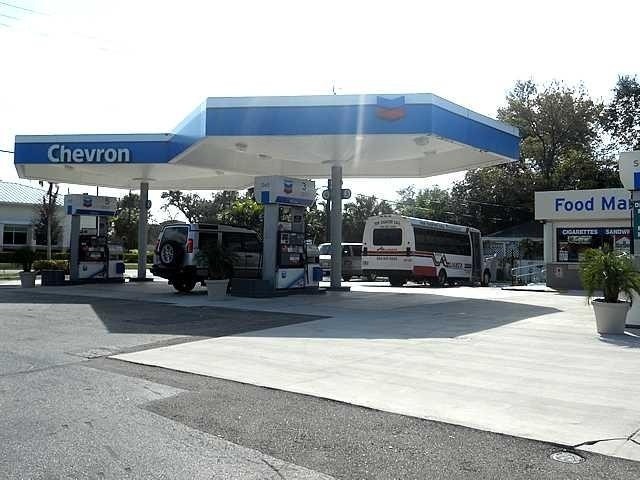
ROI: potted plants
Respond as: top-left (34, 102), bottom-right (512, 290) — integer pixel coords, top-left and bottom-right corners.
top-left (191, 239), bottom-right (241, 300)
top-left (10, 246), bottom-right (39, 288)
top-left (578, 247), bottom-right (640, 335)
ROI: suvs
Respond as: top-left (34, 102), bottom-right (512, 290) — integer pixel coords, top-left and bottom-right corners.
top-left (152, 222), bottom-right (265, 292)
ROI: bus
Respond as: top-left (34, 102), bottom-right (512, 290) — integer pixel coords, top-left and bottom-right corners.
top-left (361, 213), bottom-right (491, 287)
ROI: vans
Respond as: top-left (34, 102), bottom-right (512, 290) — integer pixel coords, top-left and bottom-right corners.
top-left (317, 243), bottom-right (379, 282)
